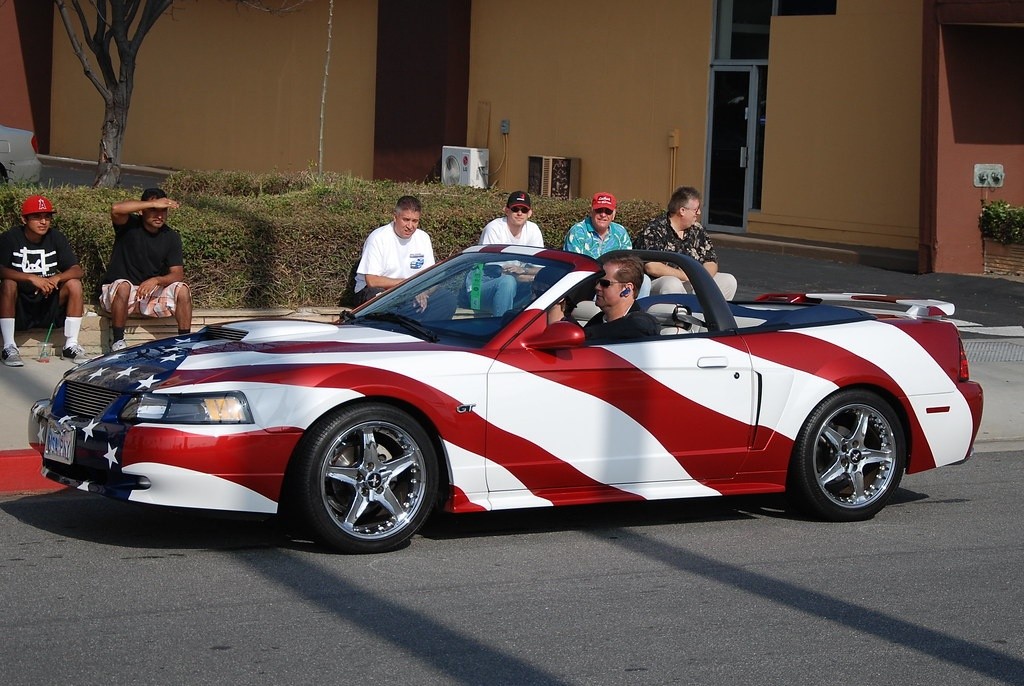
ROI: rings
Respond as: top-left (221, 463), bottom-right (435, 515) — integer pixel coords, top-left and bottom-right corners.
top-left (169, 202), bottom-right (172, 205)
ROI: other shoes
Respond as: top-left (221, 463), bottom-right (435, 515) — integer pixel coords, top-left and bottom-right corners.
top-left (111, 340), bottom-right (126, 352)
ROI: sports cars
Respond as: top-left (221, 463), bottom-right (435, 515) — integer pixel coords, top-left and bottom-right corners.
top-left (27, 242), bottom-right (985, 556)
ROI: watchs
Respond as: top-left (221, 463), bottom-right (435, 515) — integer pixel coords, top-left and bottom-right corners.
top-left (522, 267), bottom-right (529, 275)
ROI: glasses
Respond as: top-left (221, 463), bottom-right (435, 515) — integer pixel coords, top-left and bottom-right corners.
top-left (682, 206), bottom-right (700, 215)
top-left (511, 206), bottom-right (530, 213)
top-left (529, 288), bottom-right (544, 297)
top-left (595, 208), bottom-right (615, 215)
top-left (596, 278), bottom-right (630, 288)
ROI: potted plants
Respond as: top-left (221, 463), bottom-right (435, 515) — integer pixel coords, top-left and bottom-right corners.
top-left (977, 198), bottom-right (1024, 276)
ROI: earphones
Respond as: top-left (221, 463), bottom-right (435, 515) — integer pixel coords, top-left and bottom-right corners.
top-left (620, 287), bottom-right (631, 297)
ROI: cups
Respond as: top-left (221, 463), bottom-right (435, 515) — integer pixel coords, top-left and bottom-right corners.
top-left (36, 342), bottom-right (54, 363)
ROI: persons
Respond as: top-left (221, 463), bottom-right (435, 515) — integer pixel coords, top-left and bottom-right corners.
top-left (353, 196), bottom-right (457, 321)
top-left (559, 251), bottom-right (663, 344)
top-left (562, 191), bottom-right (632, 262)
top-left (632, 186), bottom-right (738, 311)
top-left (0, 194), bottom-right (91, 366)
top-left (466, 190), bottom-right (546, 317)
top-left (501, 262), bottom-right (582, 328)
top-left (98, 187), bottom-right (192, 351)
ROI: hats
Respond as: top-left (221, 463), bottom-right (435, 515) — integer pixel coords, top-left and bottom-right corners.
top-left (21, 196), bottom-right (57, 214)
top-left (536, 267), bottom-right (578, 309)
top-left (507, 191), bottom-right (530, 209)
top-left (592, 192), bottom-right (617, 211)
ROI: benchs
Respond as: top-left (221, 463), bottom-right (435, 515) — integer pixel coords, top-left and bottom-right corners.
top-left (571, 301), bottom-right (767, 337)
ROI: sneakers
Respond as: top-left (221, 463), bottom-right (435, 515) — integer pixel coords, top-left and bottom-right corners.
top-left (61, 345), bottom-right (89, 365)
top-left (1, 345), bottom-right (24, 365)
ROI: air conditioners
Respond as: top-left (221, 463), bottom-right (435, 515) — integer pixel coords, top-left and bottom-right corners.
top-left (441, 146), bottom-right (490, 189)
